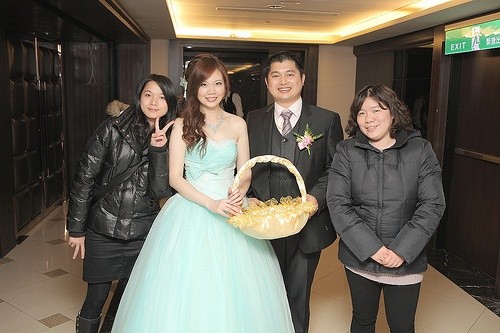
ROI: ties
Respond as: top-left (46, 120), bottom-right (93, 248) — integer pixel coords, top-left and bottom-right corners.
top-left (280, 110), bottom-right (293, 137)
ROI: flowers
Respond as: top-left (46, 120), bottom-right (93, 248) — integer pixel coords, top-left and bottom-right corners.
top-left (293, 123), bottom-right (325, 156)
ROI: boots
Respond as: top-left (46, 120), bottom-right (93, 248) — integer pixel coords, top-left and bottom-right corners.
top-left (76, 311), bottom-right (115, 333)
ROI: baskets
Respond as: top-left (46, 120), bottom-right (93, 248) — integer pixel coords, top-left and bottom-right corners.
top-left (226, 154), bottom-right (317, 240)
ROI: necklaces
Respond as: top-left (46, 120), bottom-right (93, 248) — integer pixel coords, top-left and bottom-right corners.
top-left (199, 105), bottom-right (225, 134)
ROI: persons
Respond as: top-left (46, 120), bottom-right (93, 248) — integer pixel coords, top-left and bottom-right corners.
top-left (168, 53), bottom-right (252, 332)
top-left (65, 72), bottom-right (180, 333)
top-left (243, 51), bottom-right (345, 333)
top-left (326, 83), bottom-right (446, 333)
